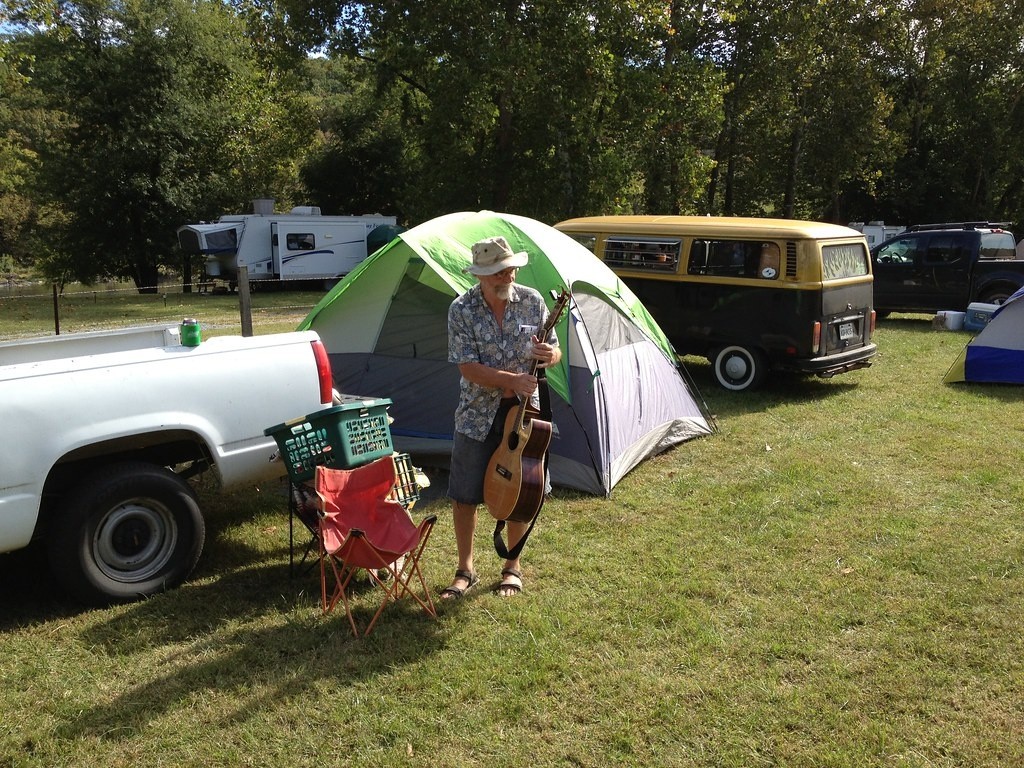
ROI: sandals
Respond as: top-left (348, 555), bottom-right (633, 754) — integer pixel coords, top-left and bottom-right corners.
top-left (440, 569), bottom-right (480, 601)
top-left (496, 567), bottom-right (522, 597)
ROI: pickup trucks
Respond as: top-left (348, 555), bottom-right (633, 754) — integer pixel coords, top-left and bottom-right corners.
top-left (869, 222), bottom-right (1024, 321)
top-left (0, 318), bottom-right (346, 605)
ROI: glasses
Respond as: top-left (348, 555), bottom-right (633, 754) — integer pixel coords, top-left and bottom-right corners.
top-left (494, 267), bottom-right (521, 280)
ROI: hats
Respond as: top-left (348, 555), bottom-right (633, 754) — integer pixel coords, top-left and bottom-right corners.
top-left (462, 237), bottom-right (528, 277)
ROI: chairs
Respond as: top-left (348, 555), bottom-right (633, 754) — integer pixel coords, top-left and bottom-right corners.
top-left (315, 456), bottom-right (438, 636)
top-left (278, 474), bottom-right (358, 584)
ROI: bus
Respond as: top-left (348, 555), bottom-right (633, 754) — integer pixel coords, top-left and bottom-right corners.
top-left (551, 213), bottom-right (877, 394)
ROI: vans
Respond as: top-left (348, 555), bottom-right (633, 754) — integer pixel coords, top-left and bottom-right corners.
top-left (177, 197), bottom-right (399, 297)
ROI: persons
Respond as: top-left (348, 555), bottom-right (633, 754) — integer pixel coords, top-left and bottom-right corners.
top-left (440, 236), bottom-right (562, 601)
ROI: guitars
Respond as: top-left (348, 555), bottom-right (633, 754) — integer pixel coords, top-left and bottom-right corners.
top-left (482, 285), bottom-right (573, 525)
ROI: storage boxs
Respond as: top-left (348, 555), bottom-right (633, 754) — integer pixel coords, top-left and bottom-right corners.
top-left (937, 311), bottom-right (966, 330)
top-left (963, 303), bottom-right (1001, 330)
top-left (262, 397), bottom-right (395, 483)
top-left (385, 454), bottom-right (421, 505)
